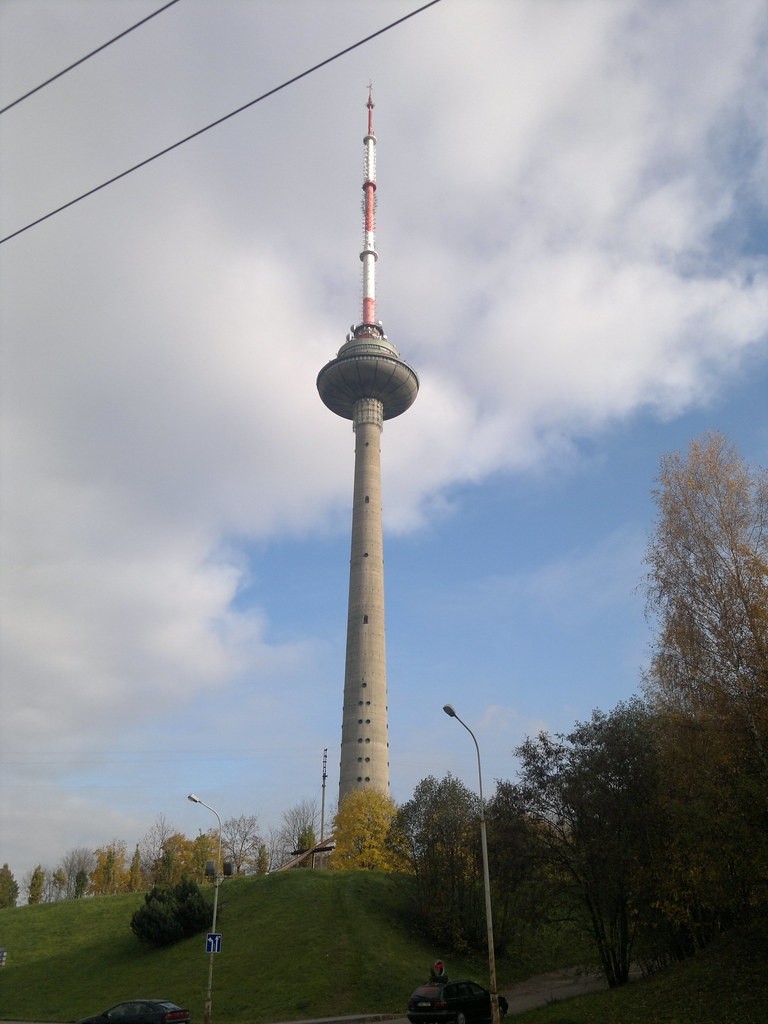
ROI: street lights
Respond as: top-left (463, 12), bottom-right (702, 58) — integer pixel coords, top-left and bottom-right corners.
top-left (443, 704), bottom-right (499, 1024)
top-left (188, 794), bottom-right (222, 1024)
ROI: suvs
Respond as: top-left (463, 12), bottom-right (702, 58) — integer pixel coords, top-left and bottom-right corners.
top-left (408, 975), bottom-right (509, 1024)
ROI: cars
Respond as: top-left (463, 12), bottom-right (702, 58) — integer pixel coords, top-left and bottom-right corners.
top-left (75, 999), bottom-right (191, 1024)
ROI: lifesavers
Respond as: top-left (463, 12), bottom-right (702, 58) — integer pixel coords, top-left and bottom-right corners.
top-left (430, 959), bottom-right (444, 976)
